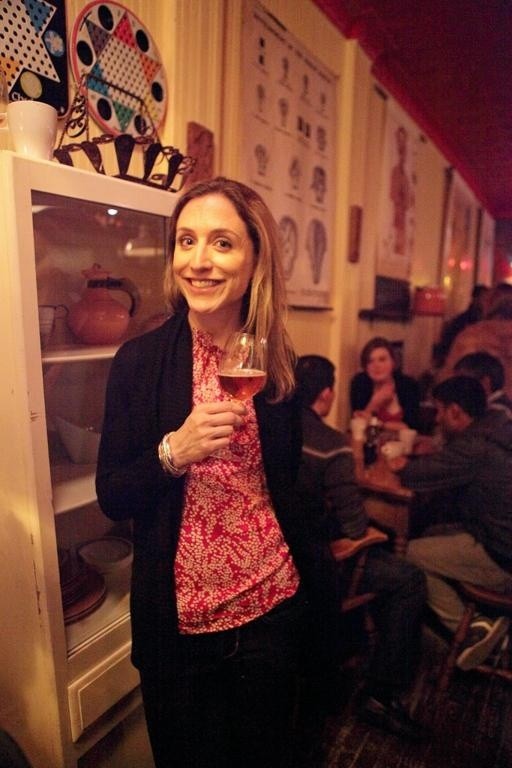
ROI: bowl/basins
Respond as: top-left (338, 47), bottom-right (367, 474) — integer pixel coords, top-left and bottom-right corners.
top-left (56, 414), bottom-right (101, 461)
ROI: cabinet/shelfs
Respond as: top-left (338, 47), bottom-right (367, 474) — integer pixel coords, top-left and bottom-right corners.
top-left (0, 149), bottom-right (180, 768)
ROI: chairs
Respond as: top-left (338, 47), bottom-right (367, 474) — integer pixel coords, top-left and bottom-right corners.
top-left (312, 527), bottom-right (389, 655)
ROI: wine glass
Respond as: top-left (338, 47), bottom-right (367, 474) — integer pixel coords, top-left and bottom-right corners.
top-left (207, 331), bottom-right (269, 466)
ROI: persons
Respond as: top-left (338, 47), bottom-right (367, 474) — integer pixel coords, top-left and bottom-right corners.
top-left (95, 179), bottom-right (312, 765)
top-left (293, 280), bottom-right (510, 691)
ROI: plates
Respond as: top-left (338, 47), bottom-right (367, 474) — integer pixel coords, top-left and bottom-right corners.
top-left (55, 536), bottom-right (133, 626)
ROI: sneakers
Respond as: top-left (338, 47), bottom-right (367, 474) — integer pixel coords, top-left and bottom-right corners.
top-left (455, 614), bottom-right (509, 671)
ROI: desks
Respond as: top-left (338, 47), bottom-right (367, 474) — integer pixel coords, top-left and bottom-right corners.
top-left (347, 469), bottom-right (457, 685)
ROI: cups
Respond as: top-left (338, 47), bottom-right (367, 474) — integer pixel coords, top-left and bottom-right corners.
top-left (2, 100), bottom-right (61, 163)
top-left (37, 300), bottom-right (69, 357)
top-left (348, 411), bottom-right (420, 483)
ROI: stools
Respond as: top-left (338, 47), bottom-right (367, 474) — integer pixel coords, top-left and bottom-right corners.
top-left (435, 581), bottom-right (512, 703)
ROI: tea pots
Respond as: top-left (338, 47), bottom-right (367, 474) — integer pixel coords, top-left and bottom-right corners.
top-left (69, 262), bottom-right (143, 348)
